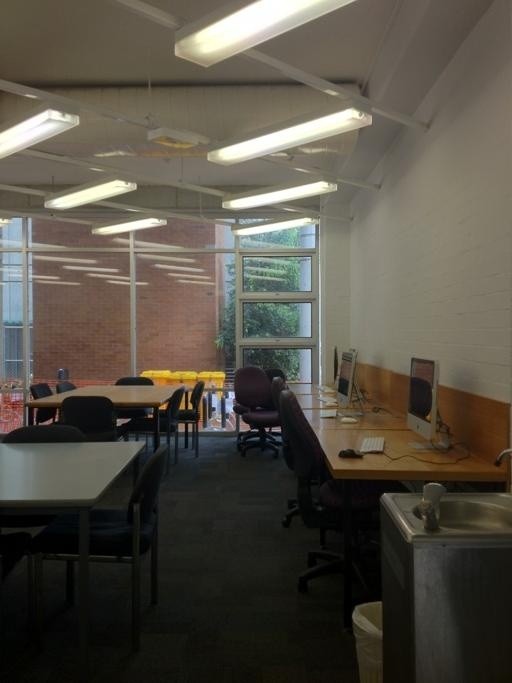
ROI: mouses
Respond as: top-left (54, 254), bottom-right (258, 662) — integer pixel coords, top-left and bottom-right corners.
top-left (325, 389), bottom-right (335, 394)
top-left (338, 448), bottom-right (362, 459)
top-left (326, 402), bottom-right (338, 407)
top-left (341, 417), bottom-right (358, 424)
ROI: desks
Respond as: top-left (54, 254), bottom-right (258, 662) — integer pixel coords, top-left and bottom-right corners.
top-left (1, 441), bottom-right (149, 655)
top-left (286, 384), bottom-right (506, 481)
top-left (28, 384), bottom-right (183, 449)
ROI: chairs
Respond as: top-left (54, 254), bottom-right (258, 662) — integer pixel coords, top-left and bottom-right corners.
top-left (238, 368), bottom-right (285, 443)
top-left (233, 366), bottom-right (280, 458)
top-left (33, 443), bottom-right (169, 649)
top-left (115, 376), bottom-right (153, 386)
top-left (290, 420), bottom-right (380, 634)
top-left (124, 386), bottom-right (185, 465)
top-left (3, 425), bottom-right (85, 443)
top-left (278, 389), bottom-right (312, 528)
top-left (163, 381), bottom-right (206, 458)
top-left (56, 381), bottom-right (76, 393)
top-left (60, 395), bottom-right (117, 441)
top-left (30, 383), bottom-right (58, 425)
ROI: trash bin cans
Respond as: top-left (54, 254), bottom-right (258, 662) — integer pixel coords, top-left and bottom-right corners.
top-left (197, 370), bottom-right (225, 421)
top-left (168, 371), bottom-right (197, 410)
top-left (352, 601), bottom-right (383, 683)
top-left (140, 369), bottom-right (169, 410)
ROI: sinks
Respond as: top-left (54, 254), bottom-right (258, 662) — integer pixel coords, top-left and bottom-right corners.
top-left (379, 492), bottom-right (512, 550)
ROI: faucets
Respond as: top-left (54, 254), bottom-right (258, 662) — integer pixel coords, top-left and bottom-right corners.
top-left (418, 481), bottom-right (447, 531)
top-left (493, 448), bottom-right (512, 468)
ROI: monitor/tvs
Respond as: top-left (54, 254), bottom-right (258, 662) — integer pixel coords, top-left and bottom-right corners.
top-left (337, 352), bottom-right (357, 406)
top-left (406, 356), bottom-right (440, 442)
top-left (332, 345), bottom-right (338, 384)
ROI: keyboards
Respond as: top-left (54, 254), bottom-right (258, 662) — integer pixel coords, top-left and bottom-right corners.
top-left (316, 396), bottom-right (337, 403)
top-left (316, 385), bottom-right (331, 390)
top-left (361, 436), bottom-right (384, 453)
top-left (320, 408), bottom-right (337, 419)
